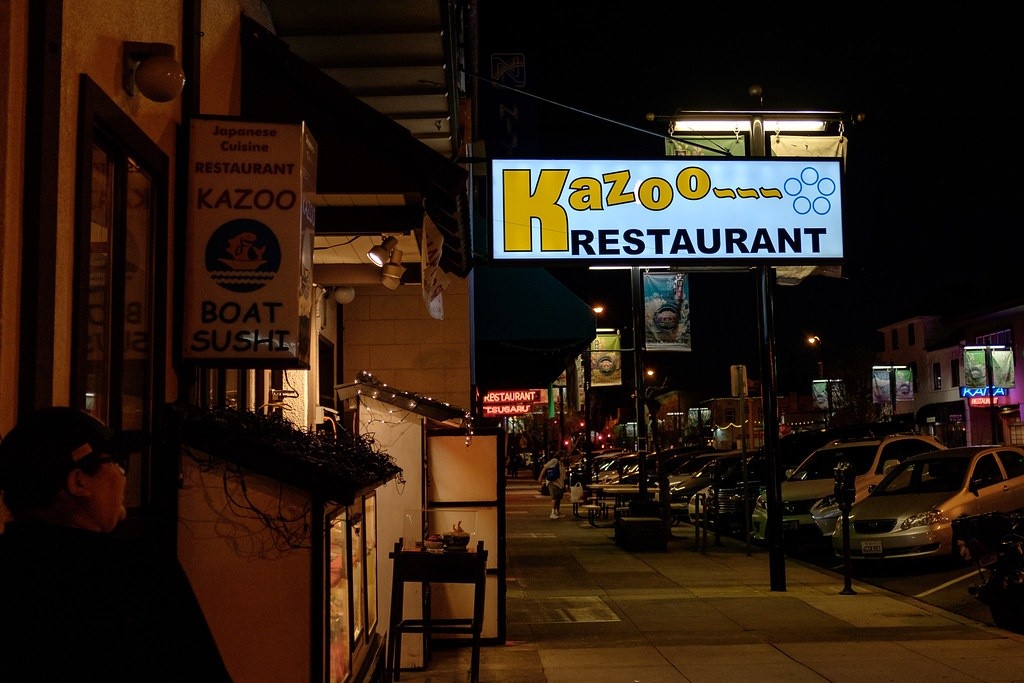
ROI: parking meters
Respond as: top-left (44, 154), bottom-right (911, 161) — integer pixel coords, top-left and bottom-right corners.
top-left (832, 459), bottom-right (857, 595)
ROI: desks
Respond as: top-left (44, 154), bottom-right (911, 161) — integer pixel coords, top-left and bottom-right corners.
top-left (586, 484), bottom-right (661, 527)
top-left (382, 538), bottom-right (488, 683)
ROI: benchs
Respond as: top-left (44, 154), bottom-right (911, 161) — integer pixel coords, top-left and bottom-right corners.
top-left (571, 496), bottom-right (686, 528)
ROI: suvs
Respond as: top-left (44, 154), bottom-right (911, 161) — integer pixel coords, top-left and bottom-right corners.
top-left (749, 428), bottom-right (955, 551)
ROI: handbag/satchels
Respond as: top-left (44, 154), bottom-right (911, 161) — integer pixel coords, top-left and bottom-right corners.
top-left (545, 460), bottom-right (560, 482)
top-left (570, 482), bottom-right (584, 503)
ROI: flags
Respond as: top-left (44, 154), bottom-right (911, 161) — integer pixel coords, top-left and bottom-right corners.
top-left (421, 215), bottom-right (450, 320)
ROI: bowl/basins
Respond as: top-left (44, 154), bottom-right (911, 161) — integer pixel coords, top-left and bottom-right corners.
top-left (424, 540), bottom-right (443, 549)
top-left (444, 535), bottom-right (470, 546)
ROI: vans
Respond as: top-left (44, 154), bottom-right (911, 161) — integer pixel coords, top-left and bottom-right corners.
top-left (716, 421), bottom-right (918, 547)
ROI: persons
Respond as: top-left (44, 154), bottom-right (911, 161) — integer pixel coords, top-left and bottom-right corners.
top-left (0, 404), bottom-right (232, 683)
top-left (537, 451), bottom-right (566, 518)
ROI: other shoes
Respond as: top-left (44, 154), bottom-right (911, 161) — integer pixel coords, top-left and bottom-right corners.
top-left (556, 512), bottom-right (566, 518)
top-left (550, 514), bottom-right (559, 519)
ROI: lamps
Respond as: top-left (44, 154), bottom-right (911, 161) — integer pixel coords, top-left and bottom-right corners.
top-left (323, 285), bottom-right (355, 305)
top-left (121, 39), bottom-right (186, 103)
top-left (368, 233), bottom-right (406, 291)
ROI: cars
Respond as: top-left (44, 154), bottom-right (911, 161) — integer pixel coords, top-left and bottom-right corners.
top-left (830, 444), bottom-right (1024, 570)
top-left (560, 446), bottom-right (757, 533)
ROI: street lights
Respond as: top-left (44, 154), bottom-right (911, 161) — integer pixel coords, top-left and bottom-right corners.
top-left (809, 335), bottom-right (824, 381)
top-left (642, 367), bottom-right (656, 391)
top-left (593, 306), bottom-right (604, 331)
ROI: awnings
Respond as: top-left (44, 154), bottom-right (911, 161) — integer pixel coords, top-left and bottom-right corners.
top-left (336, 377), bottom-right (464, 428)
